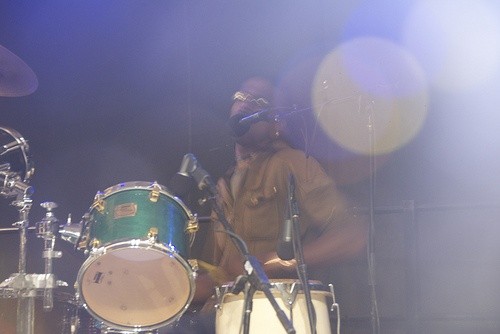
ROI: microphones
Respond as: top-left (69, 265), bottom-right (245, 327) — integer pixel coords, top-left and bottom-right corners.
top-left (230, 107), bottom-right (285, 138)
top-left (170, 152), bottom-right (201, 198)
top-left (276, 214), bottom-right (298, 262)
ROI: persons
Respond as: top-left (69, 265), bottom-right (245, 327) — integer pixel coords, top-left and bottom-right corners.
top-left (192, 73), bottom-right (372, 334)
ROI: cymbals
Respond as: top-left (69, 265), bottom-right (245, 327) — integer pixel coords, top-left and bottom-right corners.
top-left (0, 44), bottom-right (39, 99)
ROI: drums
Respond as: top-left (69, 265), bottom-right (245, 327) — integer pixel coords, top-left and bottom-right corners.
top-left (213, 278), bottom-right (332, 334)
top-left (1, 288), bottom-right (76, 334)
top-left (0, 126), bottom-right (34, 206)
top-left (70, 180), bottom-right (199, 333)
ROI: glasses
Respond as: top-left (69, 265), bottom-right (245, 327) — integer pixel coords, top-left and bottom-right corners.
top-left (232, 90), bottom-right (273, 107)
